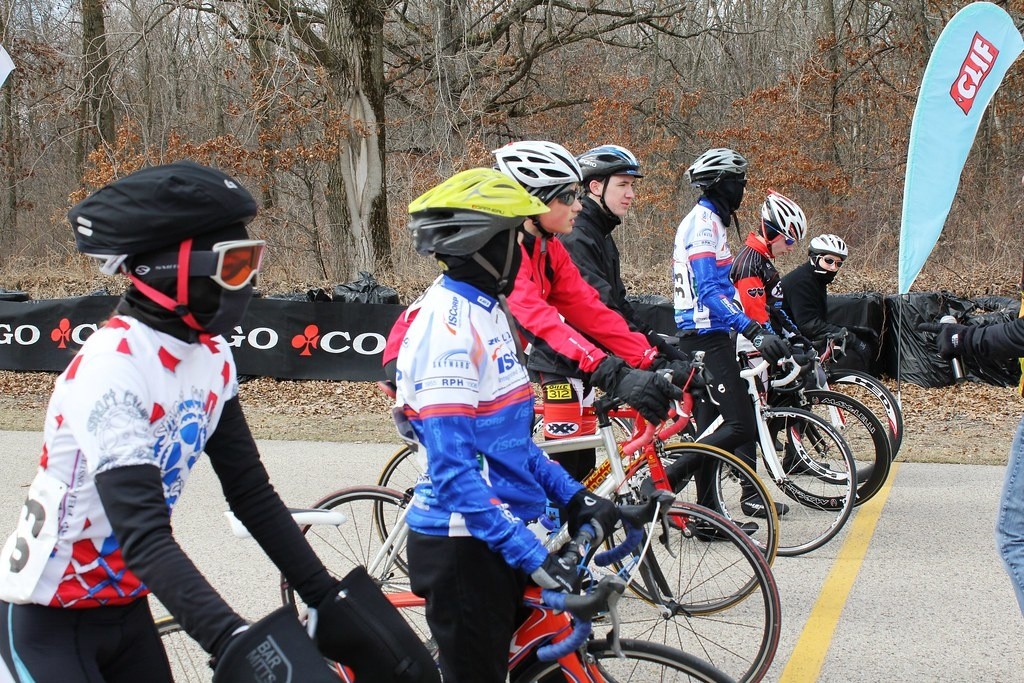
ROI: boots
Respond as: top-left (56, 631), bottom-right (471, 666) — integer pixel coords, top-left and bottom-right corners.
top-left (782, 454), bottom-right (830, 476)
top-left (740, 480), bottom-right (790, 517)
top-left (771, 428), bottom-right (784, 451)
top-left (695, 500), bottom-right (759, 542)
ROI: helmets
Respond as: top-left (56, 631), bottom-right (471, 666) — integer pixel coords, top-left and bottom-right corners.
top-left (68, 161), bottom-right (257, 334)
top-left (575, 144), bottom-right (646, 184)
top-left (808, 233), bottom-right (849, 262)
top-left (684, 147), bottom-right (750, 192)
top-left (491, 140), bottom-right (583, 204)
top-left (408, 167), bottom-right (551, 257)
top-left (763, 189), bottom-right (808, 242)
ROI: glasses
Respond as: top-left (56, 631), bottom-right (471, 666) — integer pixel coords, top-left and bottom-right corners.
top-left (784, 237), bottom-right (795, 247)
top-left (209, 240), bottom-right (265, 291)
top-left (555, 190), bottom-right (583, 206)
top-left (820, 256), bottom-right (846, 269)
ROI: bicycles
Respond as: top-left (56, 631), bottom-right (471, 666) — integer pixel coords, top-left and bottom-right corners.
top-left (371, 328), bottom-right (781, 619)
top-left (275, 348), bottom-right (783, 682)
top-left (528, 327), bottom-right (905, 557)
top-left (155, 490), bottom-right (739, 683)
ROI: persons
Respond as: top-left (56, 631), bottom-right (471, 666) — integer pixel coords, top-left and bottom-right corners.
top-left (917, 251), bottom-right (1024, 612)
top-left (395, 168), bottom-right (618, 683)
top-left (732, 188), bottom-right (814, 405)
top-left (527, 145), bottom-right (688, 483)
top-left (383, 142), bottom-right (703, 427)
top-left (0, 161), bottom-right (442, 683)
top-left (673, 147), bottom-right (789, 541)
top-left (775, 233), bottom-right (879, 476)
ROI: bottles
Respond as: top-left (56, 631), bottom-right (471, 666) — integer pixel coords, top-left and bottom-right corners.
top-left (527, 513), bottom-right (556, 547)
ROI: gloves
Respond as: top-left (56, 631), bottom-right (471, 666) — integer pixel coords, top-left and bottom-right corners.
top-left (742, 320), bottom-right (791, 366)
top-left (789, 335), bottom-right (812, 352)
top-left (918, 323), bottom-right (968, 361)
top-left (646, 331), bottom-right (691, 368)
top-left (587, 353), bottom-right (685, 427)
top-left (567, 488), bottom-right (619, 548)
top-left (845, 332), bottom-right (873, 368)
top-left (532, 554), bottom-right (581, 596)
top-left (650, 355), bottom-right (705, 390)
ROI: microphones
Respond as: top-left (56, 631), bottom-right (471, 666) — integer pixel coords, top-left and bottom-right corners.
top-left (940, 315), bottom-right (968, 381)
